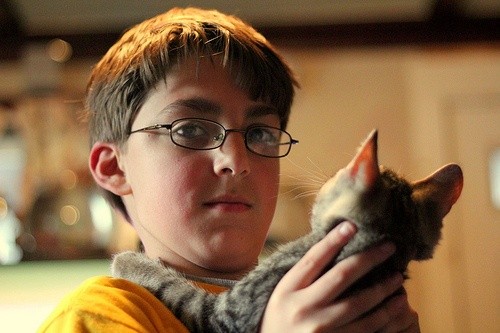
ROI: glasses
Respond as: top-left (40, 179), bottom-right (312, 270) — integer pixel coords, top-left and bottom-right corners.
top-left (127, 118), bottom-right (298, 160)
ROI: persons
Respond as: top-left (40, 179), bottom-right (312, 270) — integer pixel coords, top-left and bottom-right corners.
top-left (37, 8), bottom-right (420, 333)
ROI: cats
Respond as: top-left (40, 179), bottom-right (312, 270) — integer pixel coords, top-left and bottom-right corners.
top-left (111, 129), bottom-right (464, 333)
